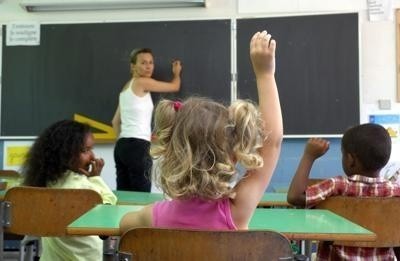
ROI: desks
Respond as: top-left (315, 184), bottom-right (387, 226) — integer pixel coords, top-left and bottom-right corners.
top-left (66, 190), bottom-right (376, 261)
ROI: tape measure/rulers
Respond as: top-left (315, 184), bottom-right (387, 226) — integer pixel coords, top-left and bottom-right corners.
top-left (73, 113), bottom-right (122, 141)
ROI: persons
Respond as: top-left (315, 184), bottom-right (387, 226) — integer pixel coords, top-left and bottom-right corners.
top-left (118, 29), bottom-right (285, 240)
top-left (111, 47), bottom-right (182, 192)
top-left (286, 122), bottom-right (400, 261)
top-left (17, 118), bottom-right (118, 261)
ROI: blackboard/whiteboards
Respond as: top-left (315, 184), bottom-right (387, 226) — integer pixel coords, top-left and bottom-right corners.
top-left (1, 12), bottom-right (361, 140)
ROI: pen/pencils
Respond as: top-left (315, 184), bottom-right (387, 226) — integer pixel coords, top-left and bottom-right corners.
top-left (88, 164), bottom-right (92, 173)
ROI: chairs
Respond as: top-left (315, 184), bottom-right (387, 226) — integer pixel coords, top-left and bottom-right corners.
top-left (3, 186), bottom-right (102, 261)
top-left (315, 195), bottom-right (400, 260)
